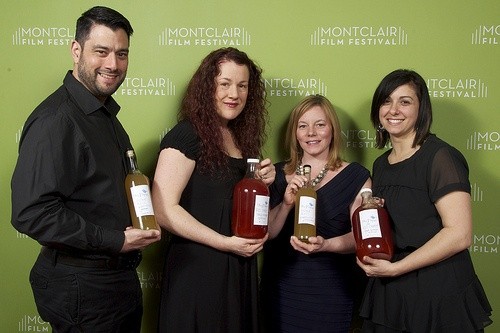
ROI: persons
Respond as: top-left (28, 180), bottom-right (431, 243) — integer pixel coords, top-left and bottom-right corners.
top-left (152, 48), bottom-right (277, 333)
top-left (257, 94), bottom-right (372, 333)
top-left (10, 6), bottom-right (161, 333)
top-left (360, 69), bottom-right (494, 333)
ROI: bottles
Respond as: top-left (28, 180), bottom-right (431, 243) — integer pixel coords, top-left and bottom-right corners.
top-left (232, 159), bottom-right (269, 239)
top-left (293, 165), bottom-right (317, 250)
top-left (124, 149), bottom-right (160, 239)
top-left (352, 189), bottom-right (393, 265)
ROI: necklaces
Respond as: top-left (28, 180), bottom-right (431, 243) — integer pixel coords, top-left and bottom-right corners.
top-left (297, 155), bottom-right (329, 187)
